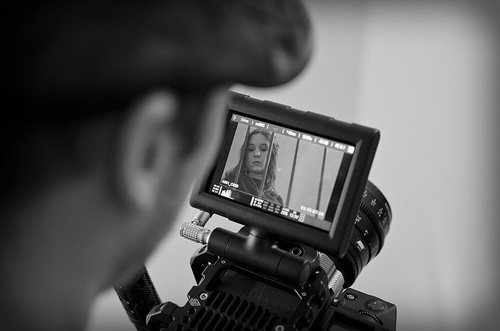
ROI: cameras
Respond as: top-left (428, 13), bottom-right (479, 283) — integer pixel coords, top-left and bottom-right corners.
top-left (145, 90), bottom-right (397, 331)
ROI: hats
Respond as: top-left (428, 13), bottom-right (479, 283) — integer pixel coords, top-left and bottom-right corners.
top-left (0, 0), bottom-right (314, 121)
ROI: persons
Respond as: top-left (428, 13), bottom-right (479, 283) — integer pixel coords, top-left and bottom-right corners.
top-left (0, 1), bottom-right (315, 330)
top-left (224, 126), bottom-right (283, 208)
